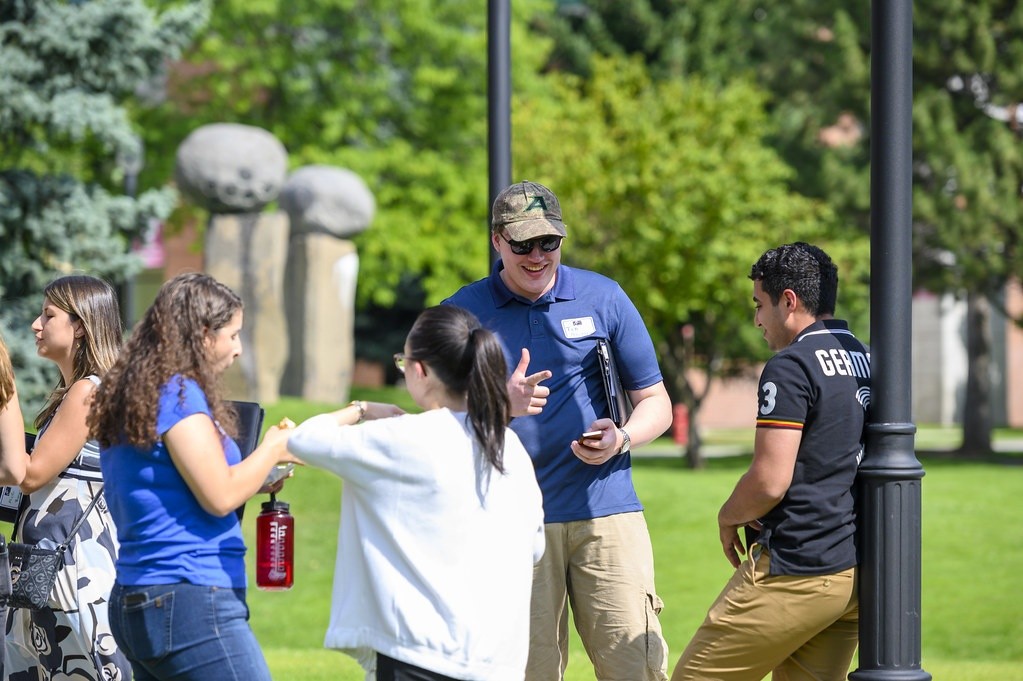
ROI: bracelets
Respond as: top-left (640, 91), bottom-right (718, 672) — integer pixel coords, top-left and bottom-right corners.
top-left (349, 400), bottom-right (365, 423)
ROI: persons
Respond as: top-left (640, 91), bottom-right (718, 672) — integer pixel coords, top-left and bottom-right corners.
top-left (440, 180), bottom-right (672, 681)
top-left (288, 305), bottom-right (546, 681)
top-left (86, 272), bottom-right (304, 681)
top-left (666, 242), bottom-right (871, 681)
top-left (0, 276), bottom-right (126, 681)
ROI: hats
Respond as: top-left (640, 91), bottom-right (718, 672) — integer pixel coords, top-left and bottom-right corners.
top-left (491, 180), bottom-right (566, 241)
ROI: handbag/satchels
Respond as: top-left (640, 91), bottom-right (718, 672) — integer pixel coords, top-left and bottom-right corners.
top-left (5, 541), bottom-right (64, 611)
top-left (0, 534), bottom-right (13, 597)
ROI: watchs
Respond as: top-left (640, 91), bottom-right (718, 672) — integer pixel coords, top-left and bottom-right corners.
top-left (618, 429), bottom-right (631, 455)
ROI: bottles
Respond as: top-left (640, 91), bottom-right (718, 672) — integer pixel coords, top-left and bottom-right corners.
top-left (256, 489), bottom-right (295, 590)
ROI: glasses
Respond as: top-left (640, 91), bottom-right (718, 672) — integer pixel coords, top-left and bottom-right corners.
top-left (497, 230), bottom-right (563, 255)
top-left (393, 352), bottom-right (428, 377)
